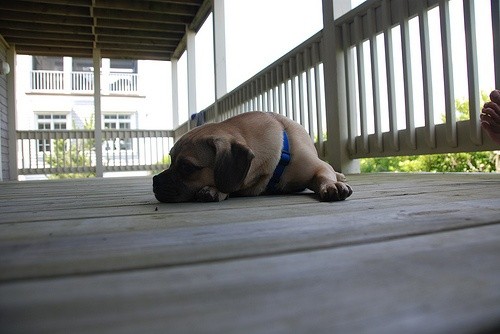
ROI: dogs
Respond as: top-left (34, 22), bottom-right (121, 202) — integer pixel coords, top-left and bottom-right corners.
top-left (152, 111), bottom-right (353, 203)
